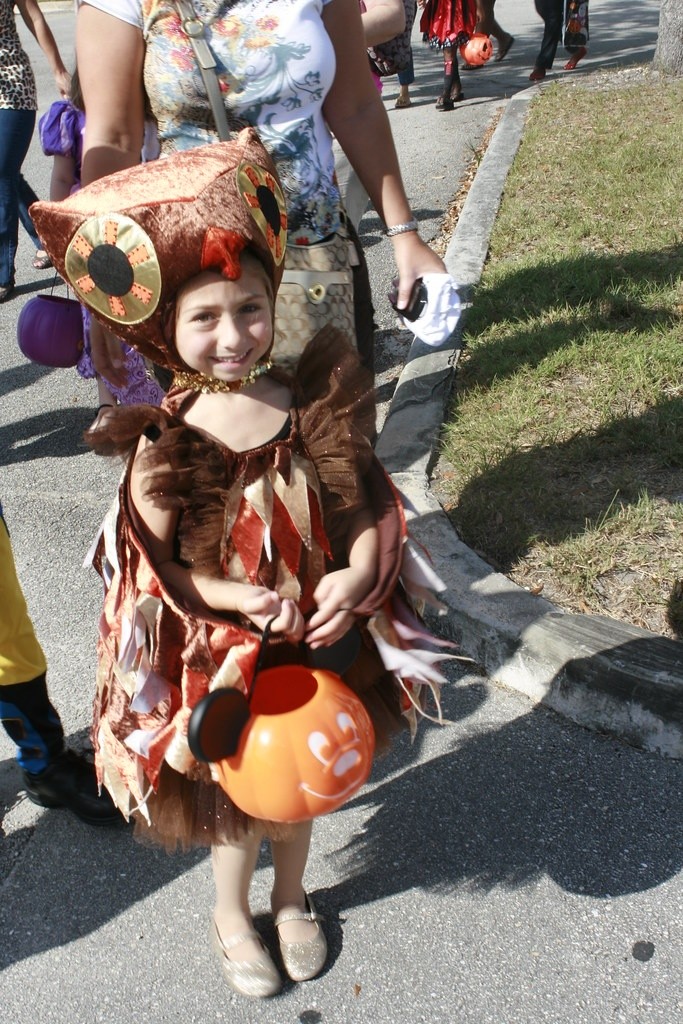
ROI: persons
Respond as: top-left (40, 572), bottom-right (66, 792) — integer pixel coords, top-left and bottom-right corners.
top-left (29, 124), bottom-right (473, 997)
top-left (1, 0), bottom-right (591, 448)
top-left (0, 504), bottom-right (122, 825)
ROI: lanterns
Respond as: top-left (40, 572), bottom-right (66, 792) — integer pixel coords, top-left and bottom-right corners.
top-left (460, 33), bottom-right (492, 65)
top-left (188, 640), bottom-right (376, 824)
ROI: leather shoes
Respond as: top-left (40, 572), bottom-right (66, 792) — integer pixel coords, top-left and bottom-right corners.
top-left (210, 892), bottom-right (330, 998)
top-left (20, 745), bottom-right (129, 826)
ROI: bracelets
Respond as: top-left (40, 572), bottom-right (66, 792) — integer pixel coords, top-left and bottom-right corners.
top-left (385, 216), bottom-right (418, 236)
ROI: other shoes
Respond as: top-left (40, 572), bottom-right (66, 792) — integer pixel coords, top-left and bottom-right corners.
top-left (494, 36), bottom-right (515, 63)
top-left (0, 284), bottom-right (14, 303)
top-left (460, 62), bottom-right (483, 70)
top-left (563, 48), bottom-right (587, 70)
top-left (435, 91), bottom-right (464, 109)
top-left (529, 68), bottom-right (546, 80)
top-left (32, 250), bottom-right (50, 269)
top-left (395, 92), bottom-right (411, 107)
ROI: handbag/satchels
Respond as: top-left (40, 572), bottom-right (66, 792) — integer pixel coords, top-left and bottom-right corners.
top-left (270, 235), bottom-right (357, 370)
